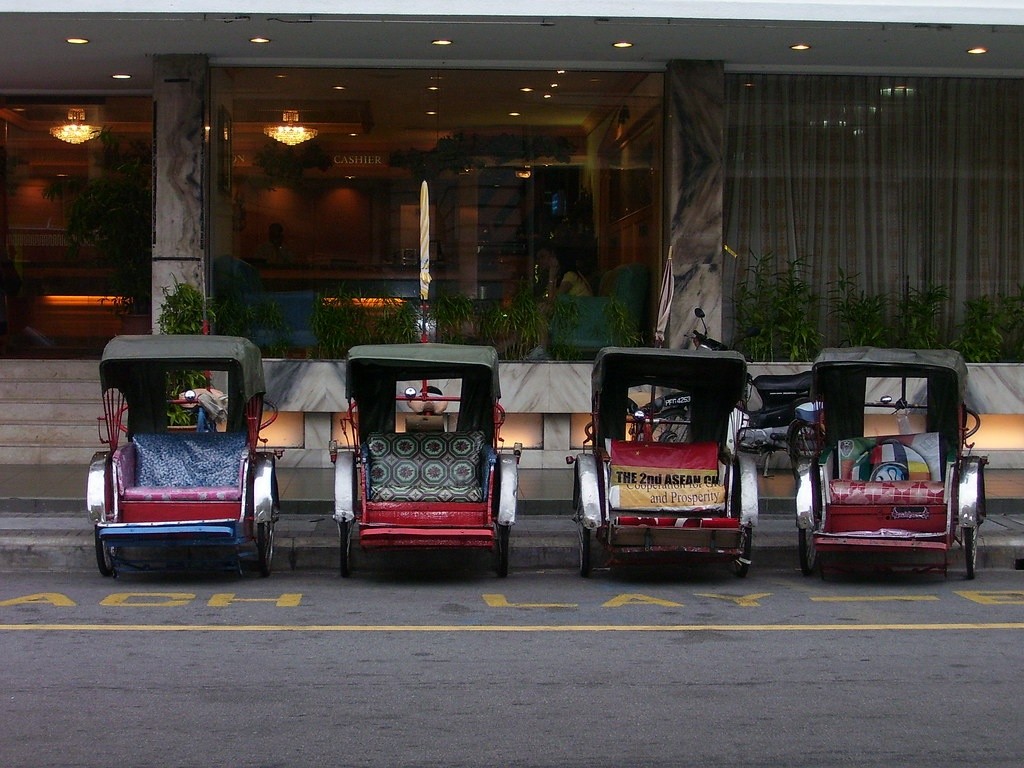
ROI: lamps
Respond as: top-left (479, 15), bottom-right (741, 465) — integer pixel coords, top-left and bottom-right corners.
top-left (50, 109), bottom-right (102, 145)
top-left (514, 167), bottom-right (531, 179)
top-left (264, 110), bottom-right (318, 147)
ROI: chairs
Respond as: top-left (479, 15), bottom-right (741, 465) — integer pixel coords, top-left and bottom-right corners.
top-left (405, 414), bottom-right (444, 433)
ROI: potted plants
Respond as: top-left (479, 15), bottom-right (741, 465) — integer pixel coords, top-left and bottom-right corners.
top-left (42, 125), bottom-right (152, 335)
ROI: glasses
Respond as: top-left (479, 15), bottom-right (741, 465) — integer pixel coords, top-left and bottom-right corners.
top-left (535, 254), bottom-right (548, 264)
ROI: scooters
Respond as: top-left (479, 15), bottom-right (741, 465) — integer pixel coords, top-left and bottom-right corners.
top-left (684, 304), bottom-right (819, 468)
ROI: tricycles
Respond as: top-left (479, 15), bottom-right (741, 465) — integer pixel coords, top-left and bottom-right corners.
top-left (84, 330), bottom-right (284, 576)
top-left (567, 343), bottom-right (759, 576)
top-left (798, 346), bottom-right (989, 581)
top-left (329, 340), bottom-right (524, 579)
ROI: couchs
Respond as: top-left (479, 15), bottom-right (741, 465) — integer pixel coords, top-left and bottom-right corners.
top-left (214, 254), bottom-right (318, 357)
top-left (551, 263), bottom-right (647, 360)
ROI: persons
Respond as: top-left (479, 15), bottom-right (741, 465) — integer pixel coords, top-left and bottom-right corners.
top-left (523, 241), bottom-right (593, 360)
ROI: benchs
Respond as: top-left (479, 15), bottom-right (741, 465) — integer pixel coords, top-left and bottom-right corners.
top-left (113, 432), bottom-right (248, 523)
top-left (601, 438), bottom-right (738, 527)
top-left (360, 432), bottom-right (495, 528)
top-left (820, 432), bottom-right (951, 532)
